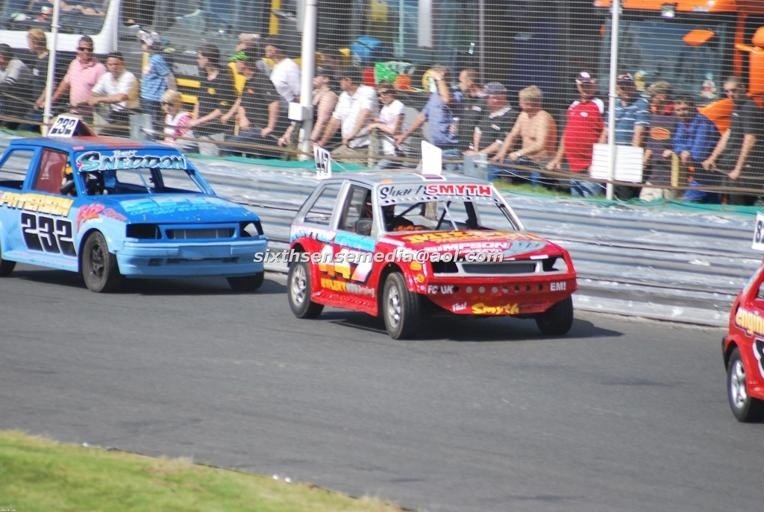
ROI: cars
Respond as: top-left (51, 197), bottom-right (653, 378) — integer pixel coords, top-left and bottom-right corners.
top-left (0, 134), bottom-right (268, 294)
top-left (722, 259), bottom-right (764, 420)
top-left (286, 171), bottom-right (577, 340)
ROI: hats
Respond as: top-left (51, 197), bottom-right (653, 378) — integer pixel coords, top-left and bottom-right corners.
top-left (617, 73), bottom-right (632, 83)
top-left (138, 29), bottom-right (160, 50)
top-left (226, 49), bottom-right (255, 62)
top-left (477, 82), bottom-right (506, 98)
top-left (575, 72), bottom-right (596, 86)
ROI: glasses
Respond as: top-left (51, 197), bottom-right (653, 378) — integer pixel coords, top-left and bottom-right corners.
top-left (376, 90), bottom-right (391, 97)
top-left (76, 46), bottom-right (94, 52)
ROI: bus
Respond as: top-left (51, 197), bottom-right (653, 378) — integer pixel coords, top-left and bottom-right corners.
top-left (1, 0), bottom-right (157, 65)
top-left (140, 0), bottom-right (389, 115)
top-left (592, 0), bottom-right (762, 139)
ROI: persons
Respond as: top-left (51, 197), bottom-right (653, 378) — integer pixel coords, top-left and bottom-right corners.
top-left (0, 26), bottom-right (763, 204)
top-left (365, 195), bottom-right (414, 231)
